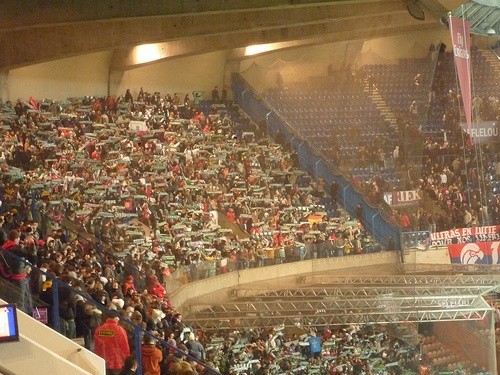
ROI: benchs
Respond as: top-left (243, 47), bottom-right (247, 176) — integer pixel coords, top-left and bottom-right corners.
top-left (0, 43), bottom-right (500, 375)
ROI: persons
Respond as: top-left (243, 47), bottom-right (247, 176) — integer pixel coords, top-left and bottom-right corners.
top-left (1, 41), bottom-right (500, 374)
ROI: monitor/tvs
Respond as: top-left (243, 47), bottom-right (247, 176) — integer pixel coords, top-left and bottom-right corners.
top-left (0, 303), bottom-right (19, 344)
